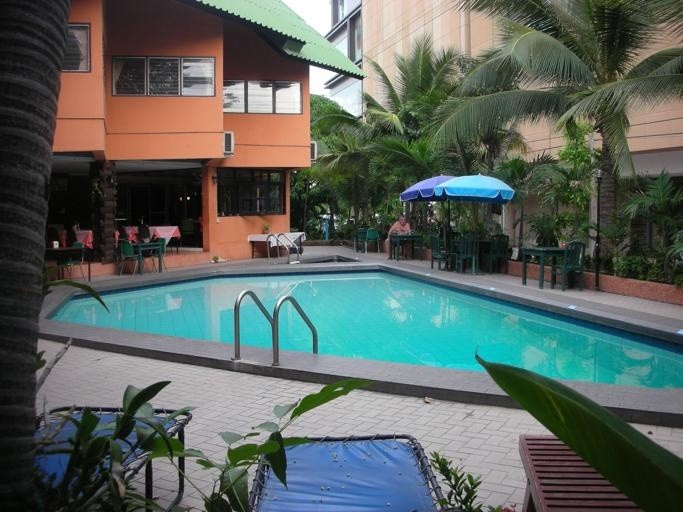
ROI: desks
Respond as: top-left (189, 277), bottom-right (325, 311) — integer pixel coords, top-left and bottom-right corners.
top-left (131, 242), bottom-right (163, 273)
top-left (247, 232), bottom-right (306, 258)
top-left (73, 231), bottom-right (120, 264)
top-left (389, 234), bottom-right (424, 260)
top-left (520, 246), bottom-right (566, 288)
top-left (45, 245), bottom-right (92, 283)
top-left (122, 224), bottom-right (182, 255)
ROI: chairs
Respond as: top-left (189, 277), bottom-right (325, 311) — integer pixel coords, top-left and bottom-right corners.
top-left (115, 239), bottom-right (139, 275)
top-left (517, 431), bottom-right (640, 512)
top-left (58, 242), bottom-right (86, 280)
top-left (394, 239), bottom-right (403, 258)
top-left (145, 235), bottom-right (167, 272)
top-left (430, 231), bottom-right (509, 275)
top-left (30, 403), bottom-right (193, 510)
top-left (551, 240), bottom-right (585, 291)
top-left (246, 433), bottom-right (453, 512)
top-left (354, 228), bottom-right (380, 254)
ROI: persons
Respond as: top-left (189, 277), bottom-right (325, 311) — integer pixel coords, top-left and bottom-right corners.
top-left (383, 214), bottom-right (412, 261)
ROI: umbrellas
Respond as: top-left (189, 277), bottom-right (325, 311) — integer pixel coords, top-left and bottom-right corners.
top-left (399, 172), bottom-right (456, 268)
top-left (434, 173), bottom-right (516, 272)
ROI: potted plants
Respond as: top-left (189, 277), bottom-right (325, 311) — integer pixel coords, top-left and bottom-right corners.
top-left (262, 224), bottom-right (271, 234)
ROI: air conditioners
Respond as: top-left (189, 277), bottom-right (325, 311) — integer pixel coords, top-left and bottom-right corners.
top-left (225, 130), bottom-right (234, 156)
top-left (311, 141), bottom-right (318, 161)
